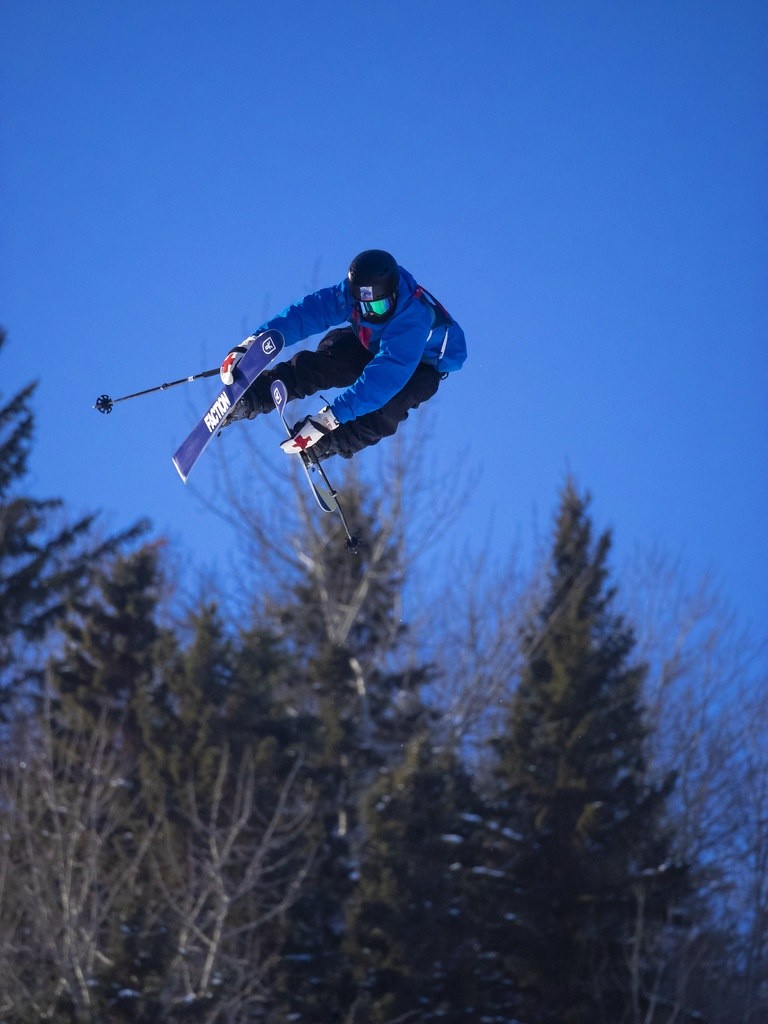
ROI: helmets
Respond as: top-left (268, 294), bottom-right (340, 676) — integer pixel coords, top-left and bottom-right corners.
top-left (348, 249), bottom-right (400, 325)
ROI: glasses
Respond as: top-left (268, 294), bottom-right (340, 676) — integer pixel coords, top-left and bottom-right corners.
top-left (351, 286), bottom-right (399, 317)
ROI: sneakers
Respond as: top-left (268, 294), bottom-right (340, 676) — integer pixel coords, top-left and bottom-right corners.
top-left (293, 421), bottom-right (338, 464)
top-left (225, 395), bottom-right (255, 421)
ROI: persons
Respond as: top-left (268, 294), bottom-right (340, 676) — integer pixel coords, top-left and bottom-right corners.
top-left (217, 250), bottom-right (467, 471)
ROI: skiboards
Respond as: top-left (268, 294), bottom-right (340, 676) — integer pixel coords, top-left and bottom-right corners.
top-left (170, 323), bottom-right (341, 516)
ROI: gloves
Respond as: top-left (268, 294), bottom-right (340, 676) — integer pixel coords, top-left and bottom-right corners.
top-left (280, 406), bottom-right (341, 454)
top-left (220, 336), bottom-right (259, 385)
